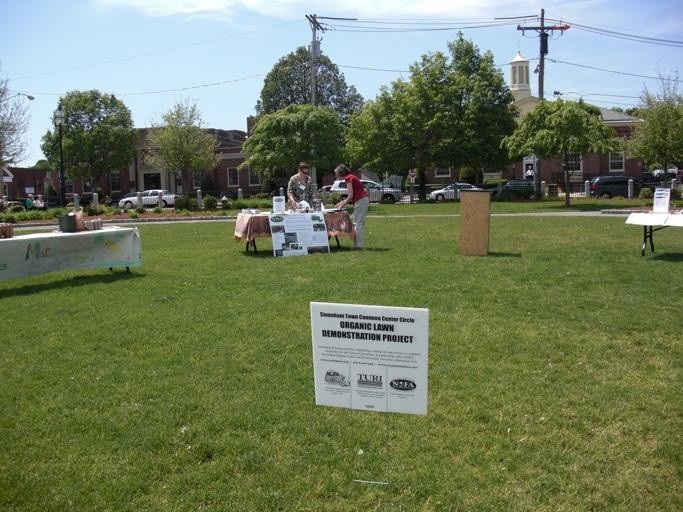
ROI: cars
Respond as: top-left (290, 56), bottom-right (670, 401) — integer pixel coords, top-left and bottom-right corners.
top-left (6, 195), bottom-right (49, 211)
top-left (430, 183), bottom-right (482, 202)
top-left (118, 189), bottom-right (180, 210)
top-left (315, 184), bottom-right (333, 195)
top-left (483, 180), bottom-right (533, 201)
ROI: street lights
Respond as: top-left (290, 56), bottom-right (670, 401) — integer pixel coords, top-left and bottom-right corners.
top-left (0, 93), bottom-right (35, 104)
top-left (53, 103), bottom-right (68, 207)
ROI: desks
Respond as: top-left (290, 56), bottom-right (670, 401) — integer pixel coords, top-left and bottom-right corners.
top-left (237, 209), bottom-right (348, 255)
top-left (632, 209), bottom-right (683, 256)
top-left (1, 227), bottom-right (138, 280)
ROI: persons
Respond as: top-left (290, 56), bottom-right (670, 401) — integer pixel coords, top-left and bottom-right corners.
top-left (286, 161), bottom-right (315, 212)
top-left (334, 162), bottom-right (370, 250)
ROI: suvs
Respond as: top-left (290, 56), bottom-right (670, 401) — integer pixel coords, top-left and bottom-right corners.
top-left (330, 180), bottom-right (404, 205)
top-left (588, 176), bottom-right (655, 200)
